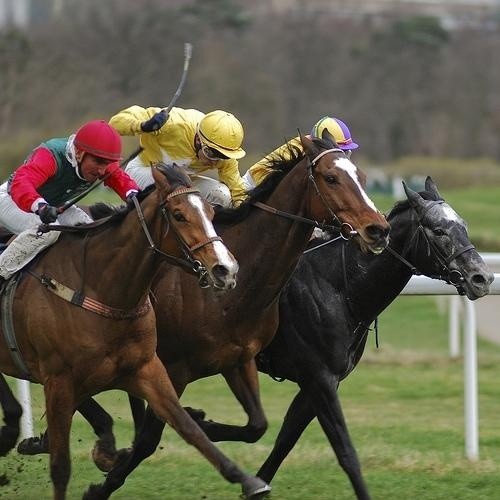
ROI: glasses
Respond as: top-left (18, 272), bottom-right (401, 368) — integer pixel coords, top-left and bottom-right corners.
top-left (198, 136), bottom-right (232, 161)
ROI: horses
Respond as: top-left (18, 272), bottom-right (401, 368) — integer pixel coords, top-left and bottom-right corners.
top-left (0, 128), bottom-right (392, 500)
top-left (0, 160), bottom-right (272, 500)
top-left (76, 174), bottom-right (495, 500)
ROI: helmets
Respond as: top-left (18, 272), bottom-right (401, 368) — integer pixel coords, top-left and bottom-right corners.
top-left (198, 110), bottom-right (246, 159)
top-left (311, 116), bottom-right (358, 150)
top-left (73, 119), bottom-right (122, 161)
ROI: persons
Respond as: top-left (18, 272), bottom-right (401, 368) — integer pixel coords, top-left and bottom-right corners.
top-left (108, 104), bottom-right (246, 209)
top-left (1, 120), bottom-right (140, 282)
top-left (241, 116), bottom-right (360, 190)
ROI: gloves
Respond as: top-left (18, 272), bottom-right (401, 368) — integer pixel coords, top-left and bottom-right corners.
top-left (141, 109), bottom-right (169, 132)
top-left (40, 204), bottom-right (58, 224)
